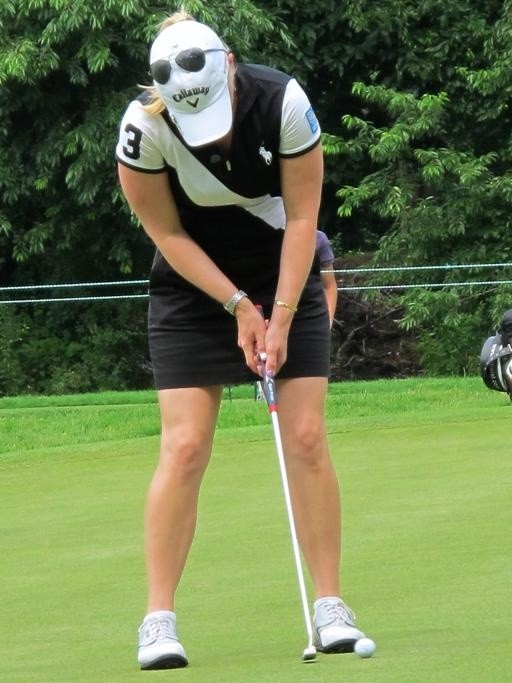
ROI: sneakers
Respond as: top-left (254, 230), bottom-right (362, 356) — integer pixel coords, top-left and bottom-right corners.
top-left (137, 616), bottom-right (188, 670)
top-left (312, 600), bottom-right (366, 653)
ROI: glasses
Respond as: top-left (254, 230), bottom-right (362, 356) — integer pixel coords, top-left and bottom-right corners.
top-left (149, 46), bottom-right (228, 85)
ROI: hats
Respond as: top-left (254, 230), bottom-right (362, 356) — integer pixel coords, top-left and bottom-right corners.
top-left (150, 20), bottom-right (232, 147)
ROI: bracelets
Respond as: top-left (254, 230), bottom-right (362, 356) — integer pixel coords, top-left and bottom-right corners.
top-left (223, 290), bottom-right (248, 316)
top-left (274, 300), bottom-right (298, 312)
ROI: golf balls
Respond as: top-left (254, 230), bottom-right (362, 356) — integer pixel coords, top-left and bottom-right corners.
top-left (352, 638), bottom-right (377, 659)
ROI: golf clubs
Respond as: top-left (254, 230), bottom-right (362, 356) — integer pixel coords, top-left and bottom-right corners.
top-left (254, 303), bottom-right (317, 665)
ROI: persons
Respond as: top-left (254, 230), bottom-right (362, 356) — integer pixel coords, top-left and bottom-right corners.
top-left (111, 12), bottom-right (368, 670)
top-left (315, 230), bottom-right (338, 330)
